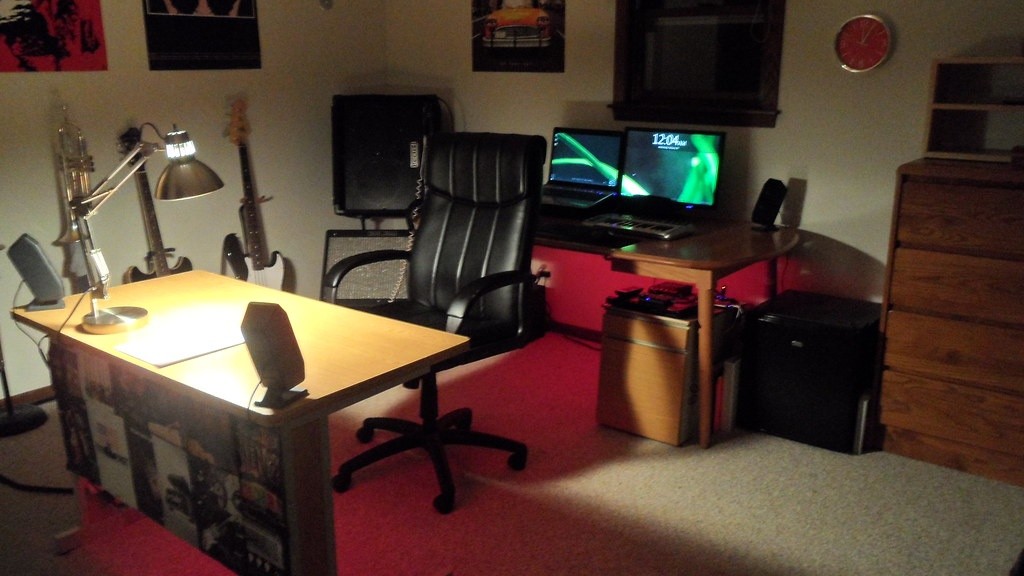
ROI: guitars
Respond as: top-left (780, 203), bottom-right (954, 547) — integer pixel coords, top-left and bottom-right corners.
top-left (218, 103), bottom-right (285, 290)
top-left (121, 126), bottom-right (193, 286)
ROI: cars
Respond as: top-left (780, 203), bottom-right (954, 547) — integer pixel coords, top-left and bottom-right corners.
top-left (480, 0), bottom-right (550, 47)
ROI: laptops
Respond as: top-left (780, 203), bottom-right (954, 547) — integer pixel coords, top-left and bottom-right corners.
top-left (538, 128), bottom-right (623, 208)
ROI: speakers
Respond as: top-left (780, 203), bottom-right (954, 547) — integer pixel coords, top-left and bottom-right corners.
top-left (751, 179), bottom-right (787, 232)
top-left (6, 233), bottom-right (66, 311)
top-left (241, 303), bottom-right (309, 409)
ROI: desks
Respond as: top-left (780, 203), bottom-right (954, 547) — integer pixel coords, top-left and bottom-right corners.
top-left (531, 212), bottom-right (800, 449)
top-left (10, 268), bottom-right (470, 576)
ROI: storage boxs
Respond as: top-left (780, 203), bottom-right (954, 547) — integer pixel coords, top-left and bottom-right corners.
top-left (737, 288), bottom-right (886, 455)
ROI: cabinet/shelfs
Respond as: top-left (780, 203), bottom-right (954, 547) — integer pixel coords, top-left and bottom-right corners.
top-left (868, 54), bottom-right (1024, 489)
top-left (596, 302), bottom-right (736, 448)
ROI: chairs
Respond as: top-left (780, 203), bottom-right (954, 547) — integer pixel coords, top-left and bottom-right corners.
top-left (321, 131), bottom-right (554, 513)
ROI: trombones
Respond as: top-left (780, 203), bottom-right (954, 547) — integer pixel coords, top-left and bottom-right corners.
top-left (52, 100), bottom-right (105, 290)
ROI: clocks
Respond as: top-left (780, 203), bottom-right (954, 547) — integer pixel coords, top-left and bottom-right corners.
top-left (833, 14), bottom-right (893, 73)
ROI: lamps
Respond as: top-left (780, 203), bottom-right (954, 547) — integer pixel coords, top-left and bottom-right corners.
top-left (66, 122), bottom-right (226, 334)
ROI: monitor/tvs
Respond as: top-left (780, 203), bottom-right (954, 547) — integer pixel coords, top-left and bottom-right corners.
top-left (619, 128), bottom-right (728, 222)
